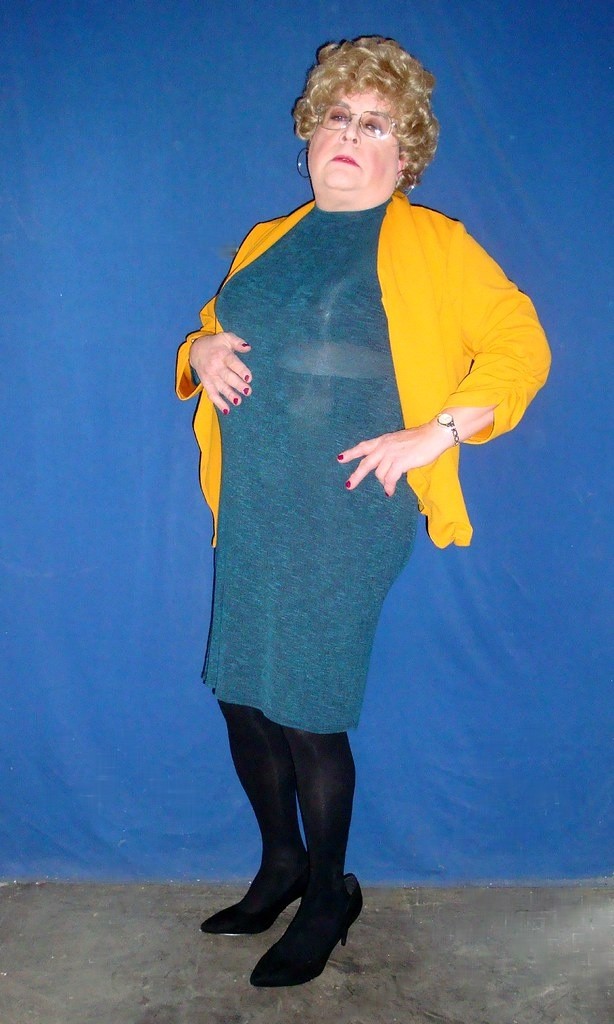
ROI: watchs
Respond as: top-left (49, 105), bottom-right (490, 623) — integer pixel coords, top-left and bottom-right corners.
top-left (435, 413), bottom-right (460, 447)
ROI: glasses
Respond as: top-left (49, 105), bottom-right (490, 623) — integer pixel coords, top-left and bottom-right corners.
top-left (317, 104), bottom-right (398, 140)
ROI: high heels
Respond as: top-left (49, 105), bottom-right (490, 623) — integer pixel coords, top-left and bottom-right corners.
top-left (200, 850), bottom-right (311, 934)
top-left (247, 873), bottom-right (364, 988)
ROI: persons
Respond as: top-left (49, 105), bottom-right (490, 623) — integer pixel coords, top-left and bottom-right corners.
top-left (174, 36), bottom-right (552, 987)
